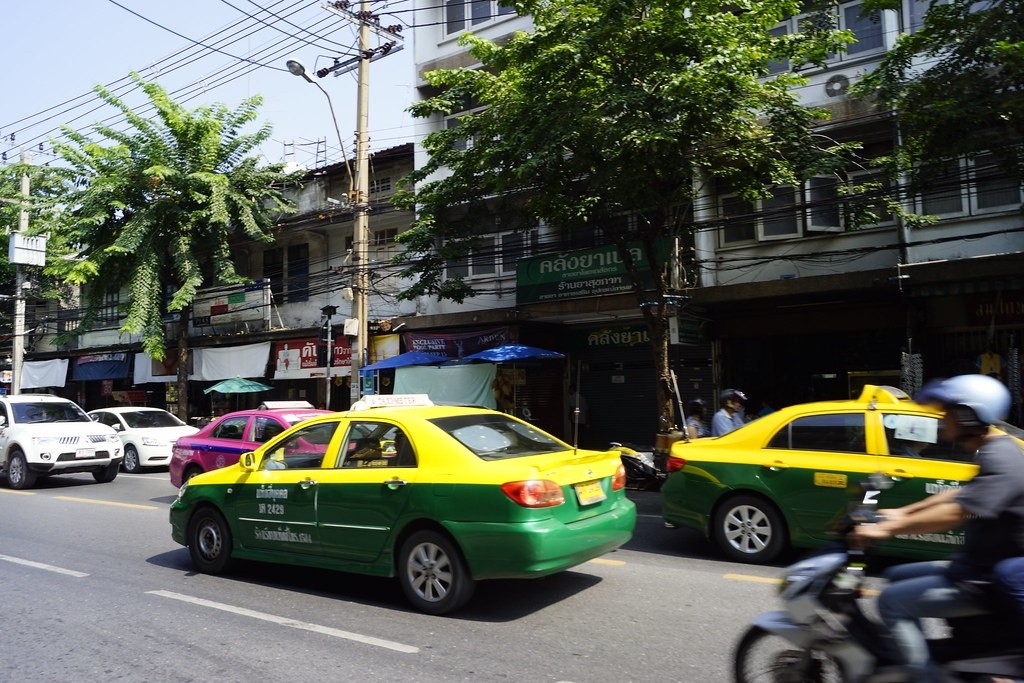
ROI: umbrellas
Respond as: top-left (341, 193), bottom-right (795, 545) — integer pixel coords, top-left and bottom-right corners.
top-left (203, 377), bottom-right (275, 411)
top-left (464, 342), bottom-right (567, 415)
top-left (357, 351), bottom-right (454, 371)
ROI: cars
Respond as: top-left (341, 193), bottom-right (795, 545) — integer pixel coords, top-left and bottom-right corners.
top-left (168, 401), bottom-right (387, 489)
top-left (659, 384), bottom-right (1024, 565)
top-left (169, 393), bottom-right (638, 614)
top-left (87, 406), bottom-right (201, 473)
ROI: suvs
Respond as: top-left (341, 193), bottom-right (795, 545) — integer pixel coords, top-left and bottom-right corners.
top-left (0, 393), bottom-right (125, 489)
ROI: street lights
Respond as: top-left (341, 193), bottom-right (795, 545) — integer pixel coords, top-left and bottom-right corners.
top-left (285, 58), bottom-right (368, 416)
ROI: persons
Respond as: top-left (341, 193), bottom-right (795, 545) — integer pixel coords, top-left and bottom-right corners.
top-left (490, 374), bottom-right (515, 412)
top-left (845, 373), bottom-right (1024, 683)
top-left (892, 440), bottom-right (930, 458)
top-left (318, 402), bottom-right (326, 410)
top-left (756, 397), bottom-right (774, 417)
top-left (214, 407), bottom-right (225, 416)
top-left (136, 414), bottom-right (147, 427)
top-left (260, 426), bottom-right (276, 442)
top-left (366, 428), bottom-right (416, 466)
top-left (684, 398), bottom-right (710, 438)
top-left (854, 434), bottom-right (865, 451)
top-left (54, 408), bottom-right (66, 418)
top-left (568, 383), bottom-right (589, 442)
top-left (22, 407), bottom-right (39, 419)
top-left (712, 388), bottom-right (748, 437)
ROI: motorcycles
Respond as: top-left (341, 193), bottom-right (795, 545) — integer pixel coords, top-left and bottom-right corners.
top-left (733, 471), bottom-right (1024, 683)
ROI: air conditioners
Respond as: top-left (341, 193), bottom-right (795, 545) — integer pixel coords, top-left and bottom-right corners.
top-left (823, 67), bottom-right (865, 100)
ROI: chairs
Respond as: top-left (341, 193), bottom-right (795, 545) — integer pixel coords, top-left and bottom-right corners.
top-left (353, 439), bottom-right (381, 461)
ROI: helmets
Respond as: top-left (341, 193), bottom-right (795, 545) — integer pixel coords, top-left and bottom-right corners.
top-left (720, 389), bottom-right (748, 402)
top-left (689, 398), bottom-right (708, 407)
top-left (916, 374), bottom-right (1011, 423)
